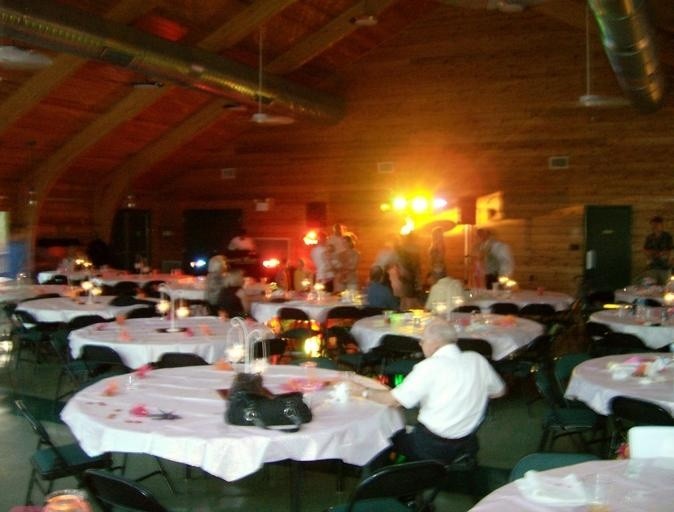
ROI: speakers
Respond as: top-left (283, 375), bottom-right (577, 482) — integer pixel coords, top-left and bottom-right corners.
top-left (306, 201), bottom-right (326, 229)
top-left (118, 209), bottom-right (151, 270)
top-left (458, 196), bottom-right (475, 224)
top-left (584, 205), bottom-right (631, 293)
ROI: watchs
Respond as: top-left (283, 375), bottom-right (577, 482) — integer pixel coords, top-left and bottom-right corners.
top-left (362, 385), bottom-right (371, 397)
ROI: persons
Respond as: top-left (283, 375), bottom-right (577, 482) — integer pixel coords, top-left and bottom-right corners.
top-left (311, 224), bottom-right (360, 293)
top-left (209, 255), bottom-right (251, 317)
top-left (343, 319), bottom-right (508, 463)
top-left (477, 227), bottom-right (512, 290)
top-left (276, 259), bottom-right (311, 292)
top-left (427, 227), bottom-right (446, 287)
top-left (645, 216), bottom-right (673, 284)
top-left (230, 230), bottom-right (256, 253)
top-left (366, 232), bottom-right (419, 311)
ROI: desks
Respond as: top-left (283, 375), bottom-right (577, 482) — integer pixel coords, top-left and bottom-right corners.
top-left (566, 353), bottom-right (674, 435)
top-left (462, 459), bottom-right (674, 512)
top-left (62, 362), bottom-right (409, 480)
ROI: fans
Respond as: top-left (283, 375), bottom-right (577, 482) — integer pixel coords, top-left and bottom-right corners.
top-left (565, 6), bottom-right (631, 109)
top-left (252, 23), bottom-right (295, 124)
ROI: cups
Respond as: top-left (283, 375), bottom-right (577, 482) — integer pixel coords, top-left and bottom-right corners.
top-left (380, 302), bottom-right (491, 336)
top-left (122, 373), bottom-right (137, 392)
top-left (42, 486), bottom-right (91, 511)
top-left (15, 272), bottom-right (28, 289)
top-left (582, 471), bottom-right (613, 509)
top-left (300, 361), bottom-right (355, 402)
top-left (616, 304), bottom-right (669, 328)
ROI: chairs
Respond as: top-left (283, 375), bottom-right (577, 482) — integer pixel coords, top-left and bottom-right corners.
top-left (555, 349), bottom-right (610, 403)
top-left (2, 267), bottom-right (674, 389)
top-left (504, 448), bottom-right (598, 489)
top-left (534, 372), bottom-right (605, 449)
top-left (447, 333), bottom-right (498, 418)
top-left (330, 456), bottom-right (451, 509)
top-left (13, 401), bottom-right (114, 512)
top-left (50, 329), bottom-right (114, 405)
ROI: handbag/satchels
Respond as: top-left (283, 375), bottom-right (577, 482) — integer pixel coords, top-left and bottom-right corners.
top-left (224, 391), bottom-right (312, 426)
